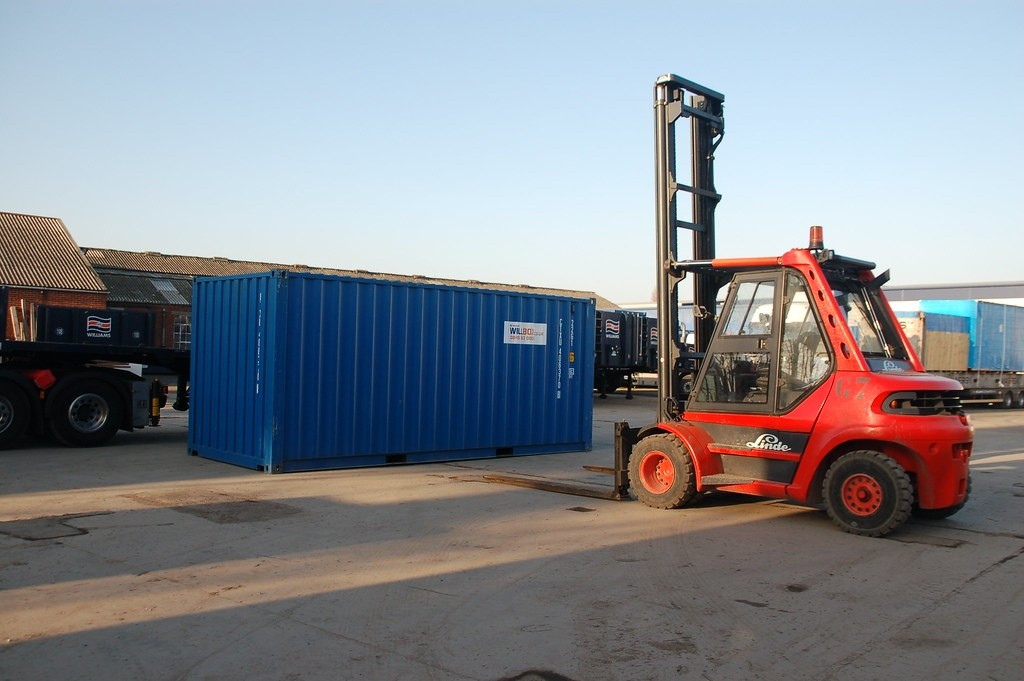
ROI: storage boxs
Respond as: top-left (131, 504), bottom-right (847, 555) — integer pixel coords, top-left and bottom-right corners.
top-left (37, 304), bottom-right (156, 347)
top-left (595, 310), bottom-right (643, 367)
top-left (634, 317), bottom-right (659, 370)
top-left (186, 269), bottom-right (596, 474)
top-left (859, 310), bottom-right (971, 371)
top-left (918, 300), bottom-right (1024, 371)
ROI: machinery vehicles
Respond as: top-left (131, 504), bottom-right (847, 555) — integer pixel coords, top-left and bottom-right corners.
top-left (481, 71), bottom-right (975, 539)
